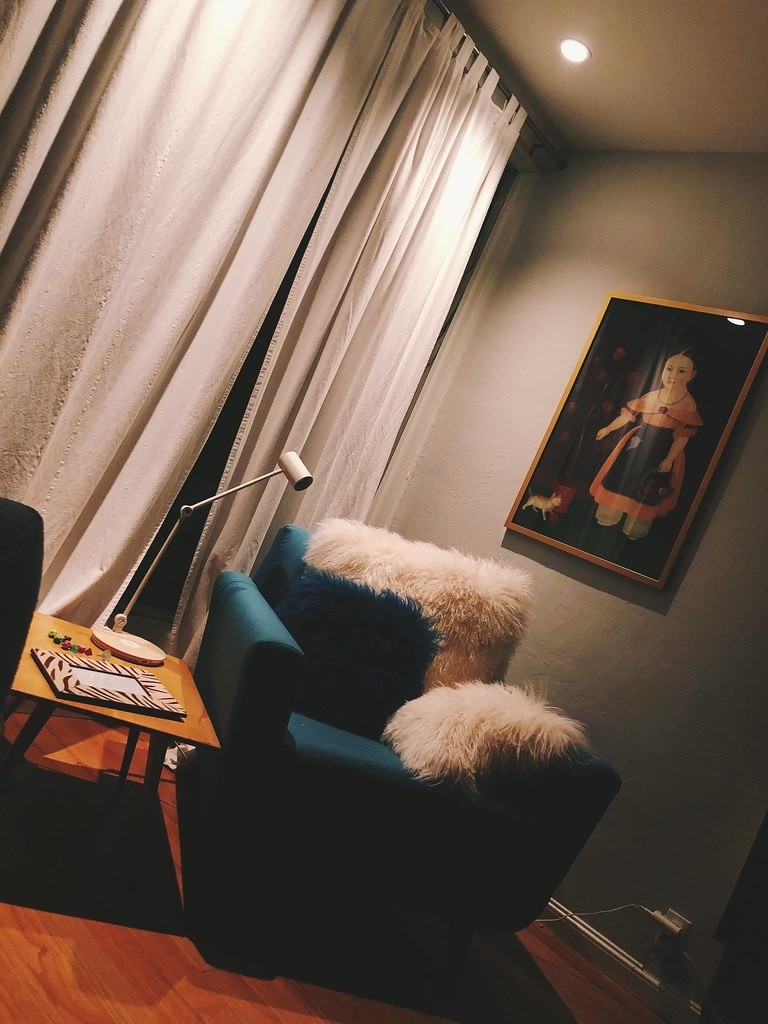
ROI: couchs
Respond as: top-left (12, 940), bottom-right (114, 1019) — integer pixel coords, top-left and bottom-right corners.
top-left (0, 497), bottom-right (45, 731)
top-left (188, 525), bottom-right (621, 933)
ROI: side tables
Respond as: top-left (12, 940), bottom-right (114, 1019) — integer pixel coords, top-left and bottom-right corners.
top-left (12, 611), bottom-right (224, 843)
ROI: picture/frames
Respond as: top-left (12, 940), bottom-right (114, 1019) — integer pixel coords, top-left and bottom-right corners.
top-left (505, 289), bottom-right (768, 590)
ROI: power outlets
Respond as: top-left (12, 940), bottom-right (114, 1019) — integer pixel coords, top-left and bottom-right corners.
top-left (653, 908), bottom-right (692, 950)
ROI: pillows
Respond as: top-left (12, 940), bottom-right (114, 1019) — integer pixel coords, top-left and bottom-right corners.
top-left (386, 679), bottom-right (595, 786)
top-left (277, 568), bottom-right (438, 741)
top-left (305, 517), bottom-right (530, 693)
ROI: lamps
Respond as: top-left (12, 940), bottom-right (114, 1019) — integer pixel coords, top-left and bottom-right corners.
top-left (92, 451), bottom-right (313, 666)
